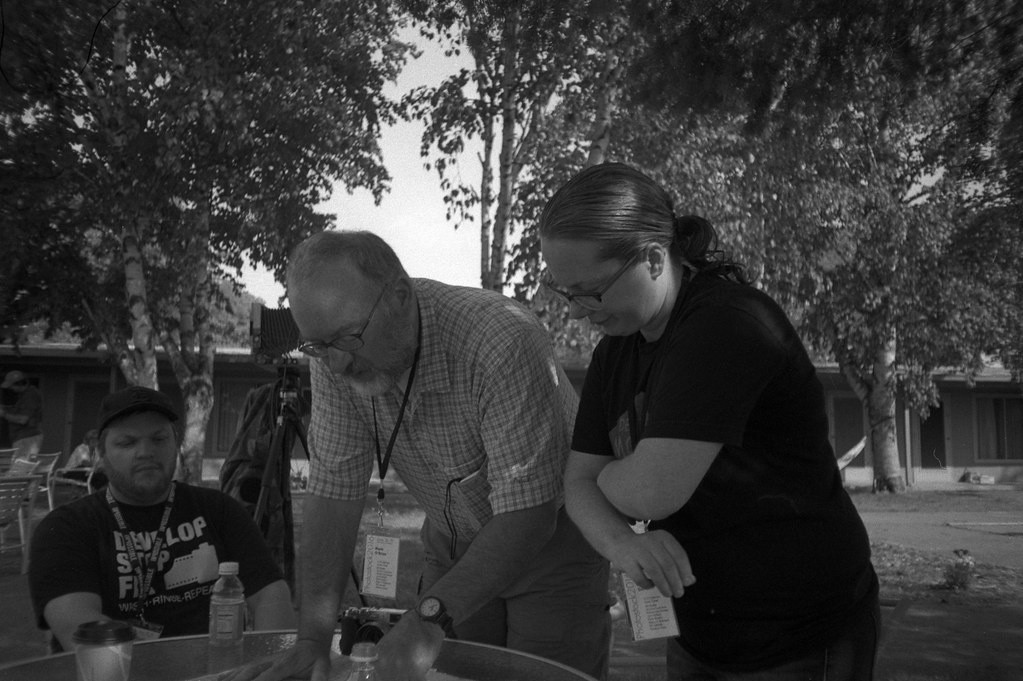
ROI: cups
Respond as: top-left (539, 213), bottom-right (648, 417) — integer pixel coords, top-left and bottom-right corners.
top-left (72, 620), bottom-right (137, 681)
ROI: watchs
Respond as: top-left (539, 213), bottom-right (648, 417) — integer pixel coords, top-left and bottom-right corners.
top-left (415, 597), bottom-right (457, 639)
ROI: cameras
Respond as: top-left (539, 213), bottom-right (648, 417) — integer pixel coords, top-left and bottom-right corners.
top-left (338, 607), bottom-right (408, 657)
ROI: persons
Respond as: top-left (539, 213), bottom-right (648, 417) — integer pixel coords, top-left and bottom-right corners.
top-left (192, 231), bottom-right (612, 681)
top-left (537, 162), bottom-right (883, 681)
top-left (0, 371), bottom-right (44, 528)
top-left (25, 385), bottom-right (298, 654)
top-left (60, 429), bottom-right (107, 492)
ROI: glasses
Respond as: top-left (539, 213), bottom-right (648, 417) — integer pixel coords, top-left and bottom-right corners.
top-left (297, 289), bottom-right (386, 357)
top-left (548, 256), bottom-right (638, 311)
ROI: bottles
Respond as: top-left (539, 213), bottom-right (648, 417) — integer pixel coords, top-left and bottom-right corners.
top-left (209, 562), bottom-right (245, 647)
top-left (339, 642), bottom-right (382, 681)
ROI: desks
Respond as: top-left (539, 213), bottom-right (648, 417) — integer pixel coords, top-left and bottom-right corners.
top-left (0, 628), bottom-right (598, 681)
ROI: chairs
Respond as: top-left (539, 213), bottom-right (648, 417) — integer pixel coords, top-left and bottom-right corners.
top-left (0, 431), bottom-right (104, 575)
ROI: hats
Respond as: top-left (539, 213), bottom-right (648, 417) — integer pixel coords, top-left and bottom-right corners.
top-left (97, 385), bottom-right (180, 439)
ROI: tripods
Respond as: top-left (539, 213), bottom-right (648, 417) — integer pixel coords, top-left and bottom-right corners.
top-left (253, 363), bottom-right (369, 606)
